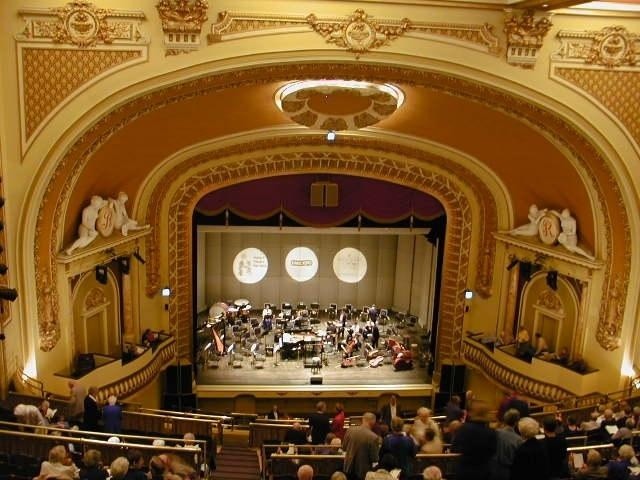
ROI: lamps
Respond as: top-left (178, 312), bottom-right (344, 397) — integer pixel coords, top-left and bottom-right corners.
top-left (269, 75), bottom-right (407, 133)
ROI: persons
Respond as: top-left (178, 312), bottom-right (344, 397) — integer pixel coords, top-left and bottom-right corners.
top-left (551, 207), bottom-right (598, 261)
top-left (517, 325), bottom-right (531, 355)
top-left (62, 191), bottom-right (104, 255)
top-left (143, 328), bottom-right (155, 347)
top-left (130, 346), bottom-right (141, 357)
top-left (496, 331), bottom-right (506, 347)
top-left (225, 303), bottom-right (381, 357)
top-left (15, 379), bottom-right (201, 479)
top-left (553, 346), bottom-right (569, 361)
top-left (107, 191), bottom-right (151, 236)
top-left (534, 331), bottom-right (549, 358)
top-left (263, 380), bottom-right (639, 479)
top-left (496, 203), bottom-right (548, 237)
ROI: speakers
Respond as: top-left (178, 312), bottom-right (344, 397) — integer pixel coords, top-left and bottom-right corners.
top-left (310, 182), bottom-right (324, 208)
top-left (164, 364), bottom-right (197, 411)
top-left (435, 364), bottom-right (466, 415)
top-left (311, 378), bottom-right (322, 385)
top-left (325, 182), bottom-right (339, 208)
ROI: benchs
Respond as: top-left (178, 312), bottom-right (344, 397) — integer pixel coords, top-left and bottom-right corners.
top-left (0, 382), bottom-right (640, 480)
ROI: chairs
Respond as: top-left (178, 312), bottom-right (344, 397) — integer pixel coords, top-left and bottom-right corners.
top-left (198, 295), bottom-right (437, 374)
